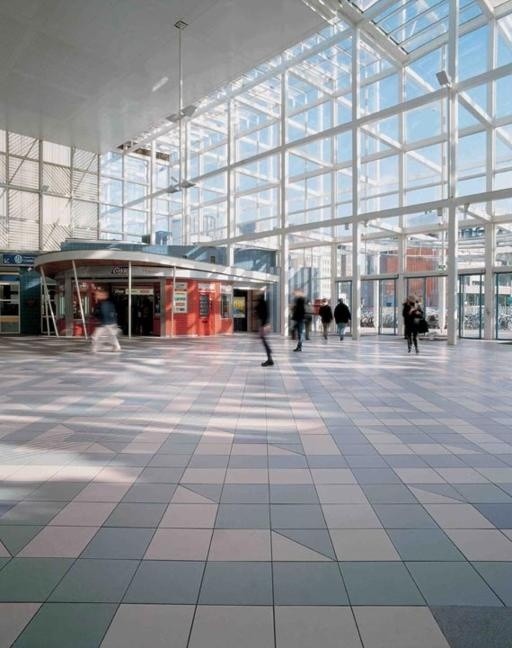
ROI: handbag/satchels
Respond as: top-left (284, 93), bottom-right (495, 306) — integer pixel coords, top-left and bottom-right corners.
top-left (418, 318), bottom-right (428, 333)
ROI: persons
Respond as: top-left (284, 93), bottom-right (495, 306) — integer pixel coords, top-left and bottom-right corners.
top-left (402, 293), bottom-right (424, 354)
top-left (319, 298), bottom-right (333, 339)
top-left (88, 290), bottom-right (122, 353)
top-left (303, 299), bottom-right (316, 340)
top-left (290, 289), bottom-right (307, 351)
top-left (91, 290), bottom-right (104, 322)
top-left (333, 298), bottom-right (351, 340)
top-left (253, 291), bottom-right (275, 366)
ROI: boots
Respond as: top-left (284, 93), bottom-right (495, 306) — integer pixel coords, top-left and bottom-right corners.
top-left (294, 341), bottom-right (302, 351)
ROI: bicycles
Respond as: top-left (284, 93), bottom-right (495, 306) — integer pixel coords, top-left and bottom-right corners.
top-left (355, 307), bottom-right (512, 331)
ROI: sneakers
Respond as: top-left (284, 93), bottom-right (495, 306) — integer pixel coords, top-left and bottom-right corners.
top-left (262, 360), bottom-right (273, 366)
